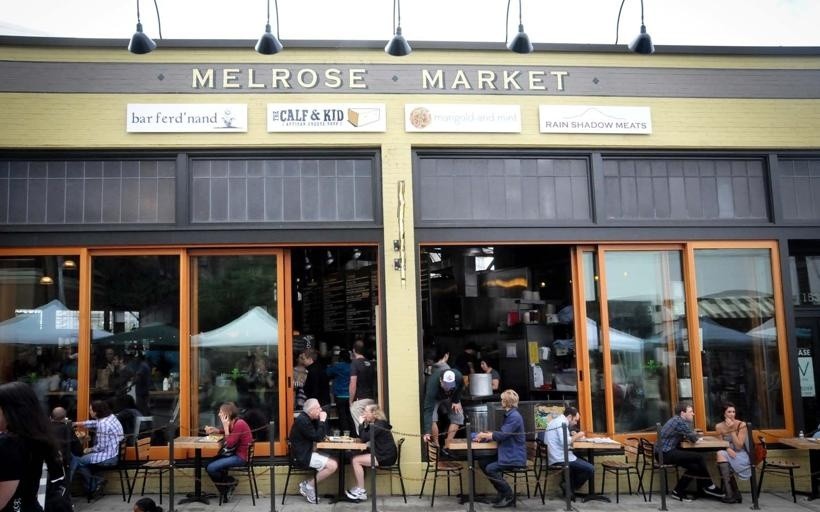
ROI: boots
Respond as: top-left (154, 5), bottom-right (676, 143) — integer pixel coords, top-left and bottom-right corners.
top-left (716, 462), bottom-right (742, 504)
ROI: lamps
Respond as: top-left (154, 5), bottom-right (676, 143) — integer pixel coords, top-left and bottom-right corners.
top-left (381, 0), bottom-right (416, 59)
top-left (126, 0), bottom-right (167, 59)
top-left (611, 0), bottom-right (655, 55)
top-left (255, 0), bottom-right (286, 57)
top-left (504, 0), bottom-right (537, 56)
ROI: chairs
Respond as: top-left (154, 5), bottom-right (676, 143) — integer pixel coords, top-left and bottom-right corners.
top-left (282, 438), bottom-right (319, 505)
top-left (637, 437), bottom-right (685, 503)
top-left (502, 438), bottom-right (545, 507)
top-left (85, 437), bottom-right (132, 502)
top-left (757, 436), bottom-right (801, 504)
top-left (217, 439), bottom-right (259, 506)
top-left (127, 436), bottom-right (176, 505)
top-left (364, 437), bottom-right (408, 504)
top-left (601, 437), bottom-right (648, 504)
top-left (418, 439), bottom-right (464, 508)
top-left (533, 437), bottom-right (576, 505)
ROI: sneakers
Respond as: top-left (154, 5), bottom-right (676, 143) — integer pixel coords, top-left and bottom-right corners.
top-left (299, 479), bottom-right (319, 503)
top-left (703, 486), bottom-right (726, 498)
top-left (671, 490), bottom-right (691, 502)
top-left (223, 478), bottom-right (238, 503)
top-left (91, 477), bottom-right (108, 500)
top-left (345, 489), bottom-right (367, 500)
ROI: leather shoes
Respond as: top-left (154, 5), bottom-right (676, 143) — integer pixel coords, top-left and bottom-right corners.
top-left (492, 495), bottom-right (503, 502)
top-left (493, 496), bottom-right (514, 508)
top-left (560, 481), bottom-right (575, 503)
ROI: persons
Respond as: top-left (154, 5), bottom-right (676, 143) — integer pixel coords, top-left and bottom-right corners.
top-left (543, 406), bottom-right (595, 502)
top-left (288, 398), bottom-right (339, 503)
top-left (714, 403), bottom-right (752, 503)
top-left (345, 404), bottom-right (398, 500)
top-left (205, 402), bottom-right (253, 498)
top-left (0, 381), bottom-right (48, 512)
top-left (473, 389), bottom-right (527, 507)
top-left (656, 403), bottom-right (725, 501)
top-left (420, 347), bottom-right (527, 455)
top-left (42, 399), bottom-right (124, 502)
top-left (95, 349), bottom-right (152, 415)
top-left (133, 498), bottom-right (162, 512)
top-left (287, 341), bottom-right (376, 436)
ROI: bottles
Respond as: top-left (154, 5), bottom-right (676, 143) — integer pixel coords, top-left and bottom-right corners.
top-left (66, 379), bottom-right (74, 392)
top-left (507, 300), bottom-right (520, 327)
top-left (696, 429), bottom-right (705, 442)
top-left (162, 377), bottom-right (171, 391)
top-left (798, 430), bottom-right (805, 442)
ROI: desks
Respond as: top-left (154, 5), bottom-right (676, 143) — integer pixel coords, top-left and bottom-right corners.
top-left (446, 439), bottom-right (499, 504)
top-left (778, 437), bottom-right (819, 501)
top-left (678, 435), bottom-right (729, 503)
top-left (572, 438), bottom-right (621, 503)
top-left (167, 437), bottom-right (222, 505)
top-left (316, 439), bottom-right (366, 504)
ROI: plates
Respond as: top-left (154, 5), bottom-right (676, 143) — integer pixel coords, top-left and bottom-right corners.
top-left (806, 438), bottom-right (819, 444)
top-left (505, 343), bottom-right (517, 357)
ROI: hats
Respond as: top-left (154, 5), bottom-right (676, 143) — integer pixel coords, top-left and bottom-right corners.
top-left (441, 369), bottom-right (456, 388)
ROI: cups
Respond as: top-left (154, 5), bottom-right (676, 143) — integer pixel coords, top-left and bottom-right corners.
top-left (523, 290), bottom-right (540, 301)
top-left (548, 304), bottom-right (554, 316)
top-left (344, 430), bottom-right (350, 439)
top-left (333, 428), bottom-right (341, 435)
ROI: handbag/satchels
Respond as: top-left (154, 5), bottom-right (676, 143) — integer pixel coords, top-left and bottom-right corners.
top-left (219, 441), bottom-right (238, 457)
